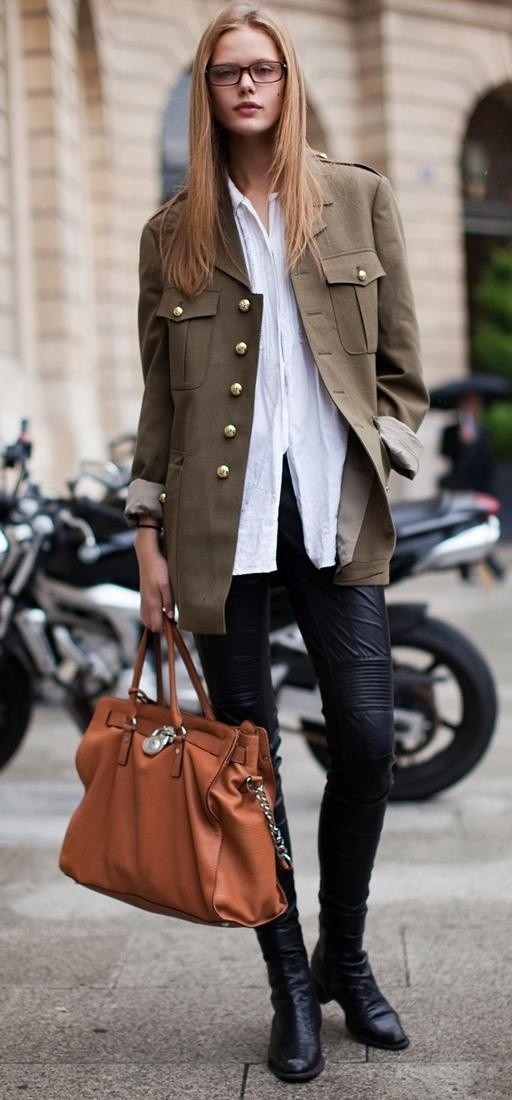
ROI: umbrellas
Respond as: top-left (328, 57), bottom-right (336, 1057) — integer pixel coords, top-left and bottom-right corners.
top-left (430, 374), bottom-right (512, 410)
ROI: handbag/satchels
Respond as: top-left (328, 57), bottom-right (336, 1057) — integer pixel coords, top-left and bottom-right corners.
top-left (56, 696), bottom-right (292, 930)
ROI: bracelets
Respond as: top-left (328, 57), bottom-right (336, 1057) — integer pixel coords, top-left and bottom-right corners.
top-left (137, 525), bottom-right (161, 532)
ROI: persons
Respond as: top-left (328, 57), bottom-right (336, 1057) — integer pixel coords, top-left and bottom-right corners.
top-left (122, 0), bottom-right (432, 1082)
top-left (437, 390), bottom-right (505, 582)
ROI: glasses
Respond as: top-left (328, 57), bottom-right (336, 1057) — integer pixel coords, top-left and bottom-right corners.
top-left (203, 61), bottom-right (286, 85)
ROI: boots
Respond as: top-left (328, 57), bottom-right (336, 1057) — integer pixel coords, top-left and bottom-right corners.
top-left (311, 825), bottom-right (409, 1052)
top-left (252, 818), bottom-right (320, 1083)
